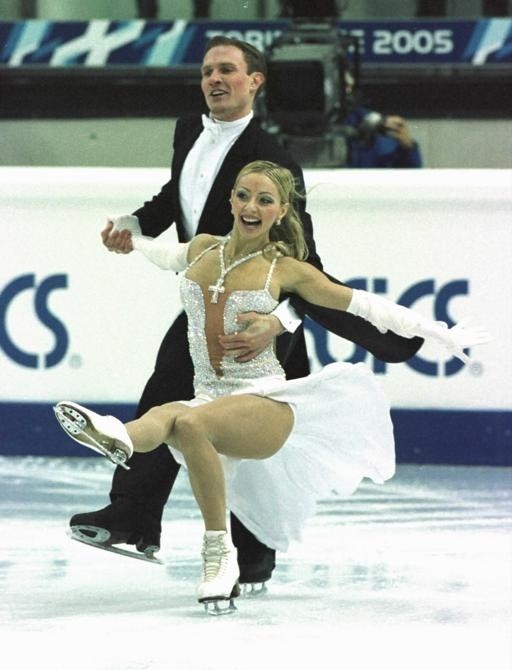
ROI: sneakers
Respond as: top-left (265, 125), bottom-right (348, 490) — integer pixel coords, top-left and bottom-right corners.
top-left (54, 400), bottom-right (134, 460)
top-left (70, 498), bottom-right (161, 554)
top-left (237, 540), bottom-right (275, 584)
top-left (197, 530), bottom-right (241, 601)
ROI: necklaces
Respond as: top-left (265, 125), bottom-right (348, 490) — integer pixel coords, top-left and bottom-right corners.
top-left (207, 236), bottom-right (275, 303)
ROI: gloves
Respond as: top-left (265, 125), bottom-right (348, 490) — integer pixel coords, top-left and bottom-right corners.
top-left (108, 214), bottom-right (189, 272)
top-left (348, 288), bottom-right (491, 364)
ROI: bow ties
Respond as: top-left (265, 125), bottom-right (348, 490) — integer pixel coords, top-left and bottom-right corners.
top-left (201, 114), bottom-right (221, 135)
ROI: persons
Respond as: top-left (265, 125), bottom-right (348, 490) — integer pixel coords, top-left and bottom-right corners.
top-left (341, 73), bottom-right (423, 168)
top-left (70, 33), bottom-right (424, 584)
top-left (53, 158), bottom-right (498, 602)
